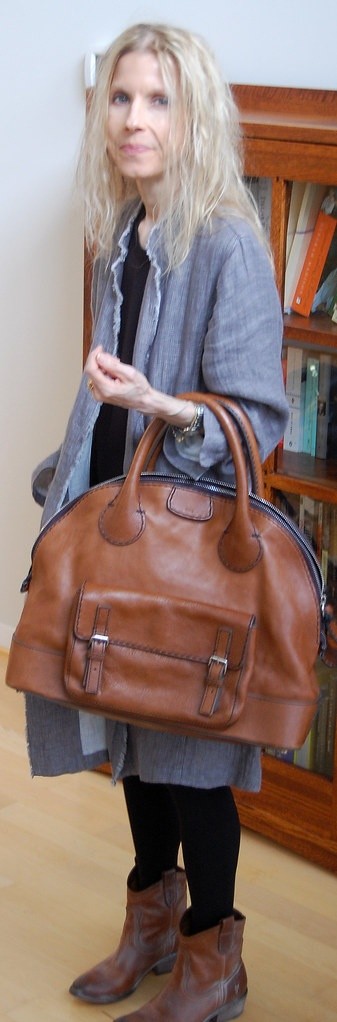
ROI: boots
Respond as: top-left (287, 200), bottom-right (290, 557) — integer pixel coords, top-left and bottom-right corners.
top-left (69, 864), bottom-right (188, 1002)
top-left (112, 904), bottom-right (248, 1022)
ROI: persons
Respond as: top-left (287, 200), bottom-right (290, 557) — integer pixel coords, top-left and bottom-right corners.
top-left (20, 22), bottom-right (290, 1022)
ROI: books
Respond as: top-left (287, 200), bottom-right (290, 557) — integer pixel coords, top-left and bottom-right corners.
top-left (236, 169), bottom-right (335, 775)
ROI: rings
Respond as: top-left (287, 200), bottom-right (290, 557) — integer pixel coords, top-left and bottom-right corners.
top-left (85, 377), bottom-right (95, 391)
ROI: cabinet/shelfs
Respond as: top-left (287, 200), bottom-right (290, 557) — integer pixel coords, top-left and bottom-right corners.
top-left (82, 85), bottom-right (336, 870)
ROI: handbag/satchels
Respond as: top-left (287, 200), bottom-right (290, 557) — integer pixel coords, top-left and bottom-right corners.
top-left (5, 392), bottom-right (330, 750)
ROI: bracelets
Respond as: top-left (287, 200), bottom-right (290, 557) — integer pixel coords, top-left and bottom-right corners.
top-left (171, 400), bottom-right (204, 443)
